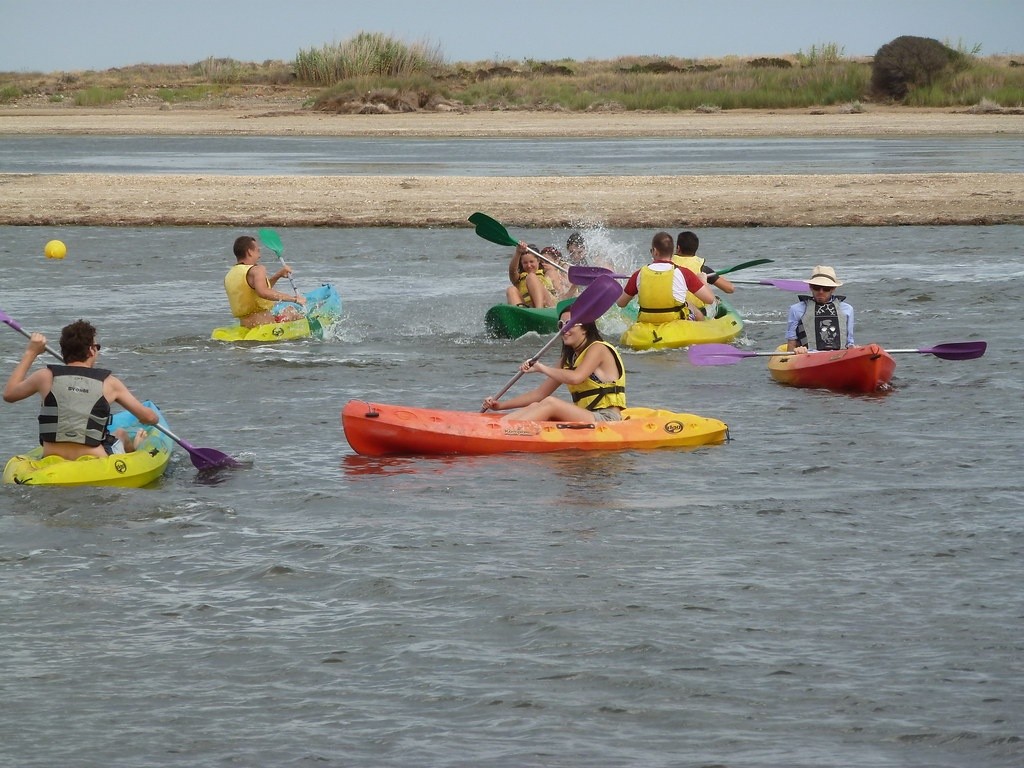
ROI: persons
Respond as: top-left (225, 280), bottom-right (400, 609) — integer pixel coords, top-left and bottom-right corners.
top-left (3, 318), bottom-right (158, 462)
top-left (223, 236), bottom-right (307, 329)
top-left (505, 231), bottom-right (613, 309)
top-left (783, 264), bottom-right (861, 355)
top-left (615, 232), bottom-right (714, 324)
top-left (481, 302), bottom-right (626, 421)
top-left (671, 232), bottom-right (735, 319)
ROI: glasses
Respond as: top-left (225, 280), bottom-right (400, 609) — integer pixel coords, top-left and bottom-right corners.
top-left (810, 285), bottom-right (834, 292)
top-left (88, 344), bottom-right (100, 351)
top-left (650, 249), bottom-right (654, 253)
top-left (558, 320), bottom-right (582, 330)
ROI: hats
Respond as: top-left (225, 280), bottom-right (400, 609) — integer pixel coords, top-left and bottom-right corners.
top-left (802, 265), bottom-right (843, 287)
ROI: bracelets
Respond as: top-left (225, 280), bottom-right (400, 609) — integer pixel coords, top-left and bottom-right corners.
top-left (293, 296), bottom-right (297, 303)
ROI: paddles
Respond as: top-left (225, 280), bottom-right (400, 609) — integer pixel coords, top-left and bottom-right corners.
top-left (479, 274), bottom-right (624, 414)
top-left (1, 309), bottom-right (236, 470)
top-left (686, 340), bottom-right (988, 368)
top-left (468, 210), bottom-right (639, 322)
top-left (567, 266), bottom-right (811, 292)
top-left (259, 226), bottom-right (324, 341)
top-left (555, 259), bottom-right (776, 318)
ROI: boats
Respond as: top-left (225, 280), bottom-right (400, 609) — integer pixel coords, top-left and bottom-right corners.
top-left (210, 282), bottom-right (343, 341)
top-left (768, 344), bottom-right (897, 394)
top-left (1, 400), bottom-right (175, 487)
top-left (342, 397), bottom-right (730, 460)
top-left (484, 296), bottom-right (744, 352)
